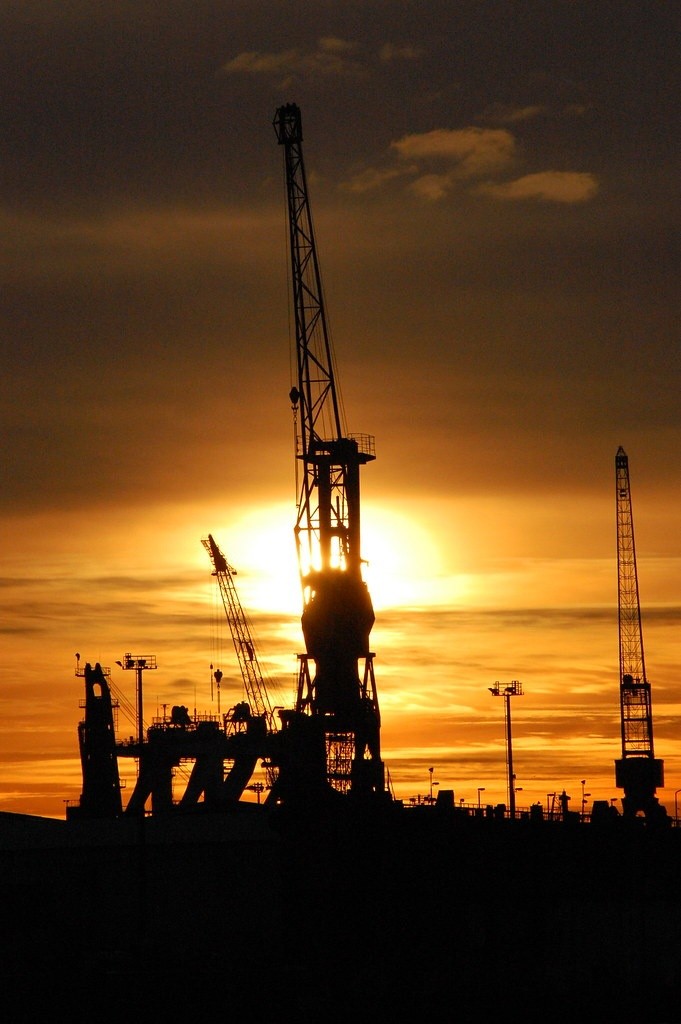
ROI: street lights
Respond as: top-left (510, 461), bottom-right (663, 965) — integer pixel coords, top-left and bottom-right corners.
top-left (478, 788), bottom-right (485, 808)
top-left (611, 799), bottom-right (617, 806)
top-left (547, 794), bottom-right (554, 812)
top-left (582, 793), bottom-right (591, 815)
top-left (431, 782), bottom-right (439, 798)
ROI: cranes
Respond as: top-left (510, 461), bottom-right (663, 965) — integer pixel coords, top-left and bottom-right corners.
top-left (202, 533), bottom-right (280, 787)
top-left (614, 444), bottom-right (657, 758)
top-left (272, 100), bottom-right (383, 794)
